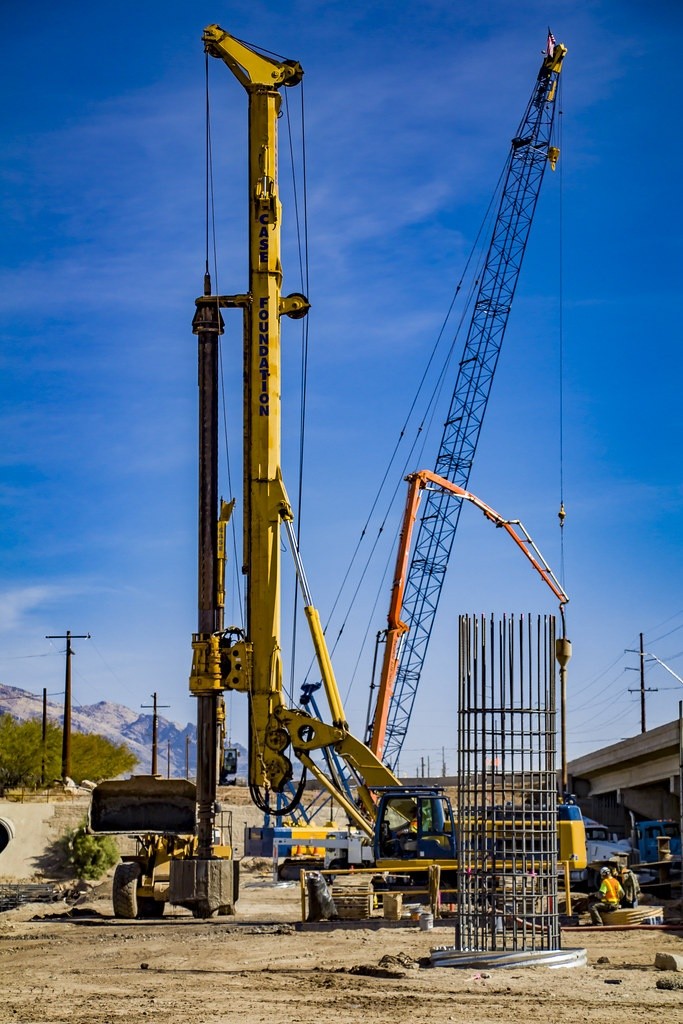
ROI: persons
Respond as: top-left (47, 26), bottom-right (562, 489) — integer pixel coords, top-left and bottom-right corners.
top-left (588, 867), bottom-right (622, 926)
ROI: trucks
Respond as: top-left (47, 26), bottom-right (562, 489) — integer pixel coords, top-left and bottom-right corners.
top-left (634, 820), bottom-right (681, 869)
top-left (579, 821), bottom-right (632, 870)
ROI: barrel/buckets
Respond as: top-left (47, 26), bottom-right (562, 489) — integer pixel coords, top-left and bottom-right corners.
top-left (420, 914), bottom-right (434, 931)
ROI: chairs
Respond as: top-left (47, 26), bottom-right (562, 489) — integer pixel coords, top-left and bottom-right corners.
top-left (400, 828), bottom-right (418, 851)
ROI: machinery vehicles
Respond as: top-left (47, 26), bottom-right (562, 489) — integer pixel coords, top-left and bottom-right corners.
top-left (87, 27), bottom-right (593, 935)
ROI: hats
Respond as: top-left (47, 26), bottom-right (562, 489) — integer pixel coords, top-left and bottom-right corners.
top-left (600, 866), bottom-right (608, 879)
